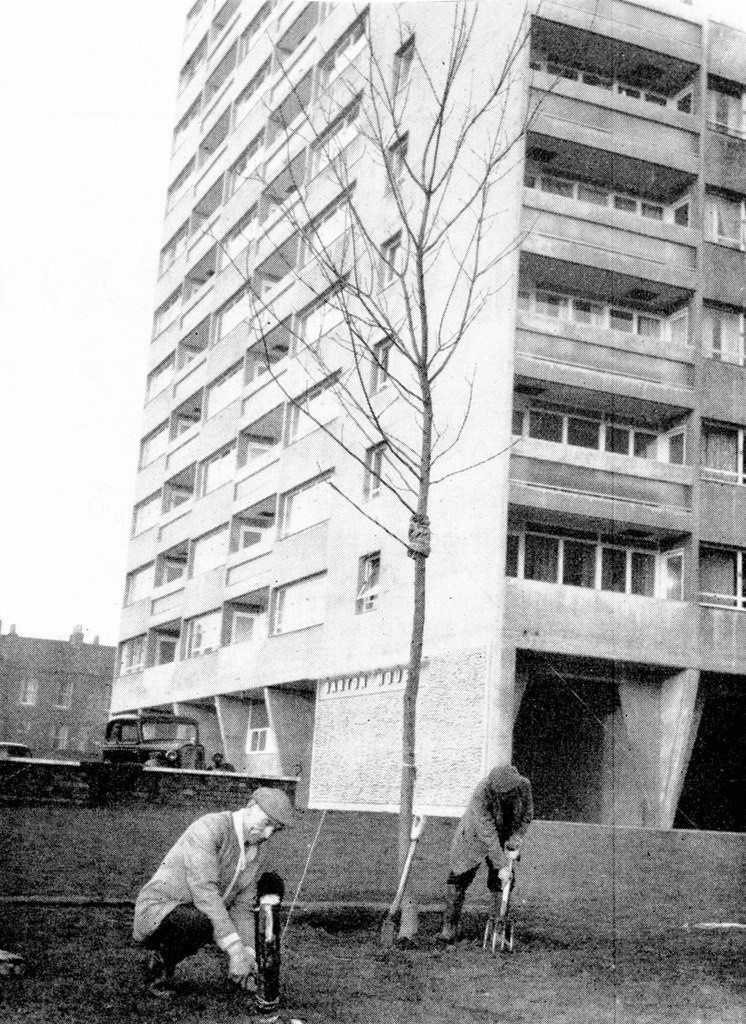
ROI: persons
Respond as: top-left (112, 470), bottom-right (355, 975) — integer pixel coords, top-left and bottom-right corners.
top-left (439, 763), bottom-right (534, 942)
top-left (205, 754), bottom-right (236, 772)
top-left (132, 787), bottom-right (297, 998)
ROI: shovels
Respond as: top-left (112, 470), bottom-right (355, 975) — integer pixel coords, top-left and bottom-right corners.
top-left (381, 814), bottom-right (428, 949)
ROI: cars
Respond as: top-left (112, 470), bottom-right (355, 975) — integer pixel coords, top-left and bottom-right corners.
top-left (0, 742), bottom-right (34, 759)
top-left (101, 706), bottom-right (214, 787)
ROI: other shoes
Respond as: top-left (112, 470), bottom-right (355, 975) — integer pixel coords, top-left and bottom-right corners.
top-left (143, 952), bottom-right (176, 998)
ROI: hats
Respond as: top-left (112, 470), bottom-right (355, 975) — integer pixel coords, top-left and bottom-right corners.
top-left (488, 765), bottom-right (522, 795)
top-left (251, 787), bottom-right (295, 830)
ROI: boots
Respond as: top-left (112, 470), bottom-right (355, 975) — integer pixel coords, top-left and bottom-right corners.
top-left (493, 893), bottom-right (511, 947)
top-left (436, 884), bottom-right (463, 941)
